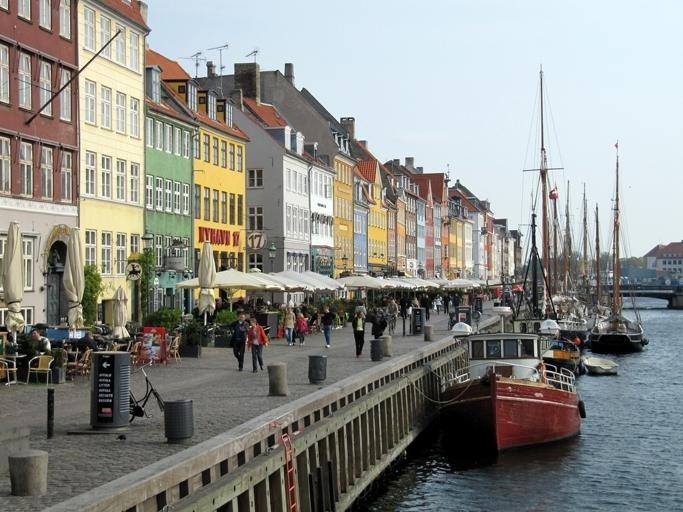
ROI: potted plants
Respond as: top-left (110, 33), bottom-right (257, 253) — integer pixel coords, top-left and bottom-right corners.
top-left (180, 310), bottom-right (237, 359)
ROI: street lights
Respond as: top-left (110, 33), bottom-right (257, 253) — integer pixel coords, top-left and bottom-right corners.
top-left (268, 243), bottom-right (277, 306)
top-left (139, 230), bottom-right (155, 319)
top-left (340, 255), bottom-right (348, 299)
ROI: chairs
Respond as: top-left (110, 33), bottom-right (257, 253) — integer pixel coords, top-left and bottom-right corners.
top-left (0, 333), bottom-right (182, 391)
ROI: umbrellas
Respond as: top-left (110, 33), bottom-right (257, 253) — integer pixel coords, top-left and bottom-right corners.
top-left (109, 284), bottom-right (130, 342)
top-left (196, 240), bottom-right (217, 324)
top-left (62, 227), bottom-right (85, 340)
top-left (1, 220), bottom-right (25, 354)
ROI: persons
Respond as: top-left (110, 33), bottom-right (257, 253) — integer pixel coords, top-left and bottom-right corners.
top-left (29, 329), bottom-right (51, 380)
top-left (246, 318), bottom-right (267, 372)
top-left (228, 311), bottom-right (249, 371)
top-left (76, 330), bottom-right (97, 360)
top-left (280, 290), bottom-right (484, 358)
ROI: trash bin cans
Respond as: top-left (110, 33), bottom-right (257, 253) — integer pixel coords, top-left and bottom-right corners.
top-left (411, 307), bottom-right (427, 336)
top-left (457, 306), bottom-right (471, 326)
top-left (89, 350), bottom-right (132, 433)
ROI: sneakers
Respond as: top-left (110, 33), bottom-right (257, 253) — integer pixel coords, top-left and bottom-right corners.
top-left (325, 345), bottom-right (331, 349)
top-left (288, 342), bottom-right (304, 346)
top-left (239, 366), bottom-right (264, 373)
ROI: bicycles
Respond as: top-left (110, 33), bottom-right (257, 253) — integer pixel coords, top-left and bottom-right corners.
top-left (127, 356), bottom-right (164, 422)
